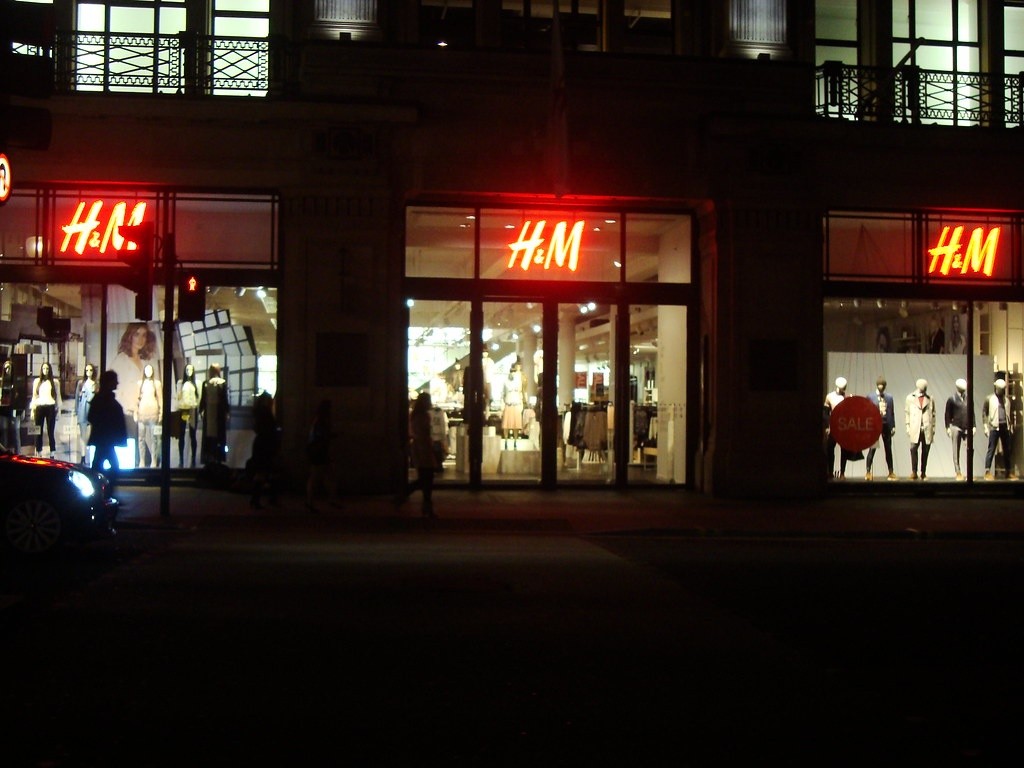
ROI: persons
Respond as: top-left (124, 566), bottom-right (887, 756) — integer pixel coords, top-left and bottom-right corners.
top-left (947, 314), bottom-right (966, 355)
top-left (177, 363), bottom-right (197, 467)
top-left (198, 363), bottom-right (230, 468)
top-left (982, 379), bottom-right (1019, 482)
top-left (247, 395), bottom-right (283, 510)
top-left (501, 362), bottom-right (526, 451)
top-left (0, 360), bottom-right (26, 455)
top-left (391, 393), bottom-right (439, 517)
top-left (111, 323), bottom-right (157, 416)
top-left (482, 348), bottom-right (496, 418)
top-left (302, 399), bottom-right (339, 512)
top-left (29, 362), bottom-right (63, 459)
top-left (944, 379), bottom-right (977, 480)
top-left (865, 377), bottom-right (899, 481)
top-left (823, 376), bottom-right (853, 480)
top-left (87, 371), bottom-right (127, 471)
top-left (928, 320), bottom-right (945, 354)
top-left (133, 365), bottom-right (163, 468)
top-left (75, 363), bottom-right (99, 465)
top-left (905, 379), bottom-right (936, 481)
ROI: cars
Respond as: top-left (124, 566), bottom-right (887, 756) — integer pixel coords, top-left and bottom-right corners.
top-left (0, 444), bottom-right (121, 562)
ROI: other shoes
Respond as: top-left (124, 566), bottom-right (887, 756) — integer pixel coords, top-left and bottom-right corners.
top-left (866, 472), bottom-right (872, 482)
top-left (839, 475), bottom-right (846, 481)
top-left (956, 475), bottom-right (965, 482)
top-left (887, 473), bottom-right (900, 480)
top-left (909, 475), bottom-right (918, 482)
top-left (1006, 473), bottom-right (1019, 480)
top-left (921, 477), bottom-right (929, 482)
top-left (984, 475), bottom-right (995, 481)
